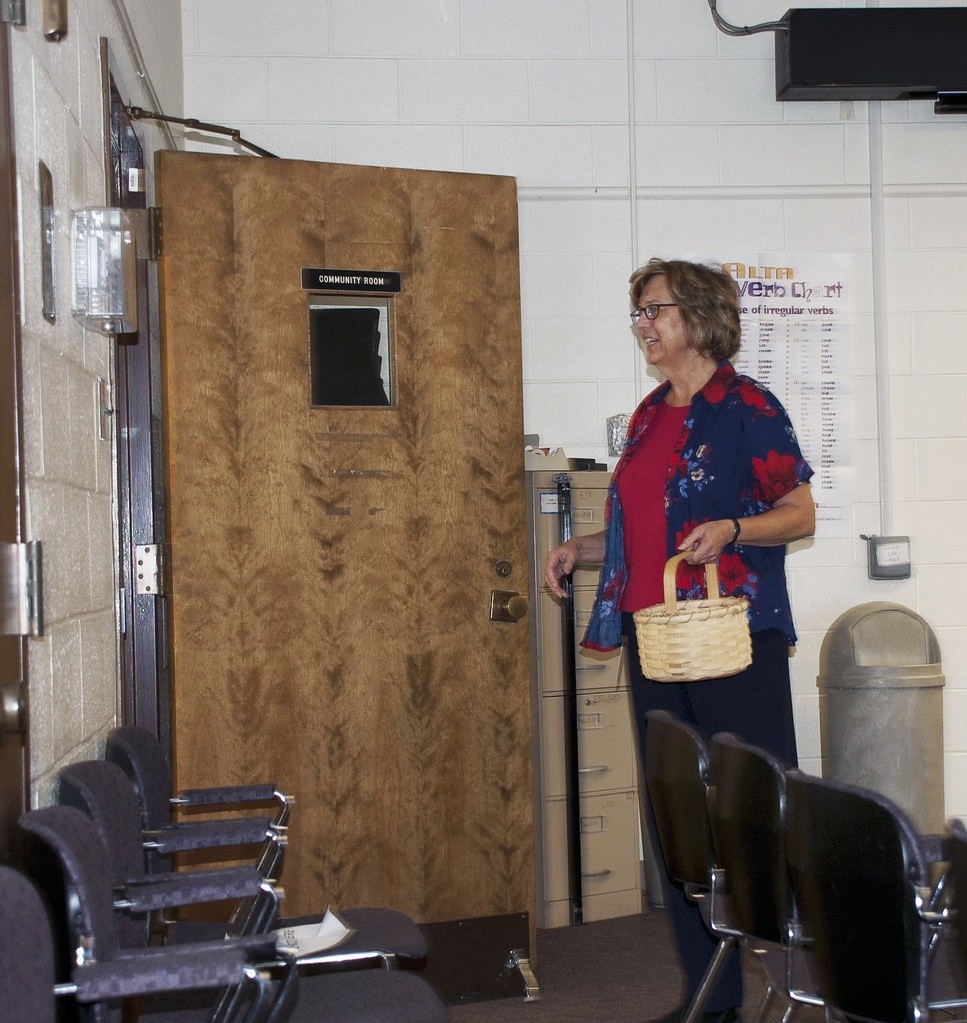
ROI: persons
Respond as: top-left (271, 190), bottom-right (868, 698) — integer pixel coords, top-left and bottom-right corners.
top-left (542, 256), bottom-right (818, 1023)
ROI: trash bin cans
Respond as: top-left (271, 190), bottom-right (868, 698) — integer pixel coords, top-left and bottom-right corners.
top-left (816, 601), bottom-right (945, 835)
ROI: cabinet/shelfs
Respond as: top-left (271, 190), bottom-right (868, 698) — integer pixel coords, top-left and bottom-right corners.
top-left (524, 471), bottom-right (642, 929)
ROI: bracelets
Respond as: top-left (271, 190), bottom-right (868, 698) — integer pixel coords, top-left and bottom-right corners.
top-left (728, 517), bottom-right (740, 545)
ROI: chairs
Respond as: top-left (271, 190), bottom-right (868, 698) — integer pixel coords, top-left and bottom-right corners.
top-left (0, 727), bottom-right (450, 1023)
top-left (644, 708), bottom-right (966, 1022)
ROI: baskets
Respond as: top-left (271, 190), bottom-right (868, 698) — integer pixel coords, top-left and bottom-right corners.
top-left (632, 551), bottom-right (753, 681)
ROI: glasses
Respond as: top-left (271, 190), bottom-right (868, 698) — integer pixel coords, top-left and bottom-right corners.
top-left (631, 304), bottom-right (681, 325)
